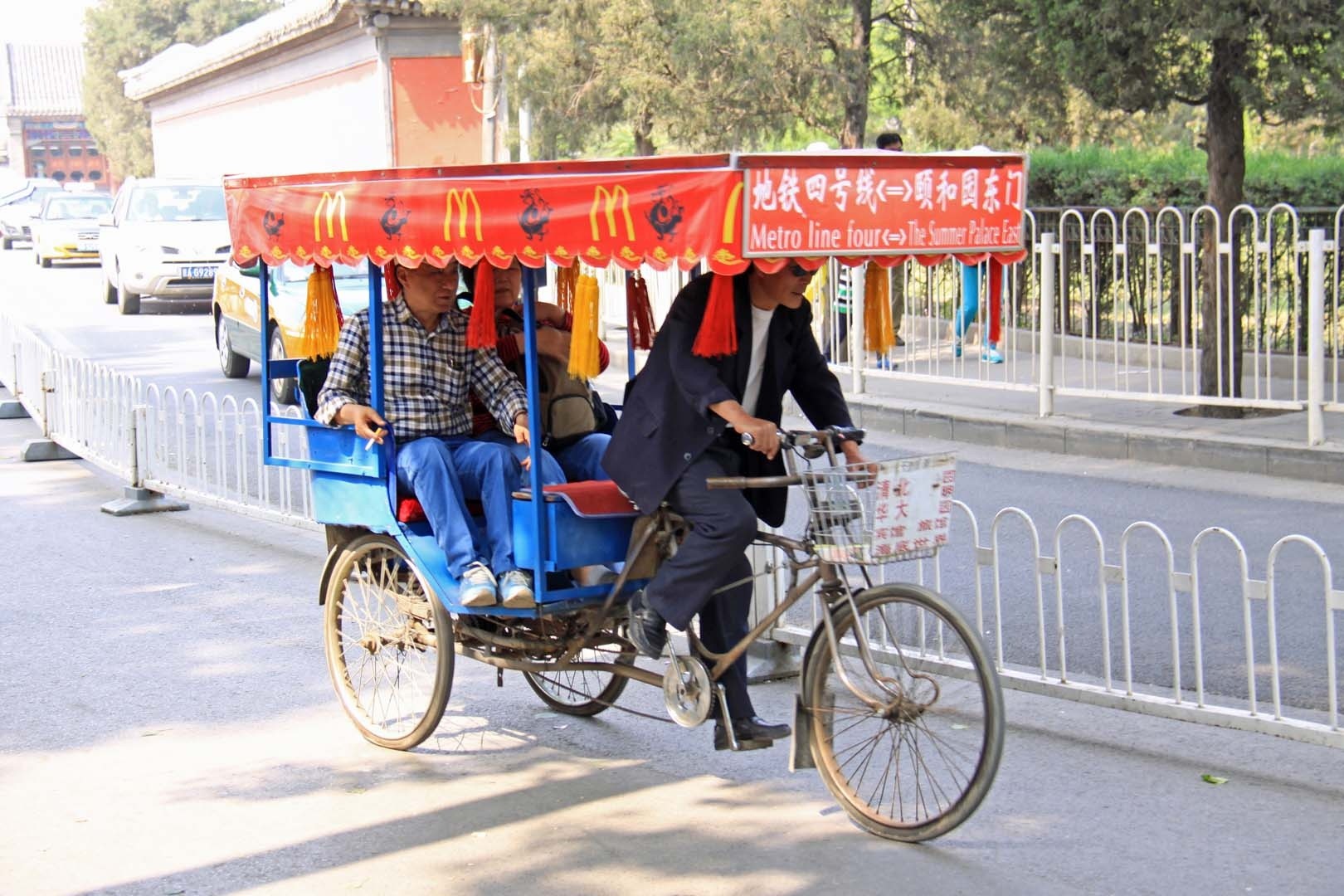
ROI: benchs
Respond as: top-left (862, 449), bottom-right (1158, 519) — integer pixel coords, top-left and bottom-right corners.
top-left (295, 354), bottom-right (425, 525)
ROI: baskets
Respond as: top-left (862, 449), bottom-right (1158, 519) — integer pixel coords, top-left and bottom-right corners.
top-left (801, 448), bottom-right (959, 568)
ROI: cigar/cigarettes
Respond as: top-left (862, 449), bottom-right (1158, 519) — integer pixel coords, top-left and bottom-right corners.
top-left (365, 427), bottom-right (382, 451)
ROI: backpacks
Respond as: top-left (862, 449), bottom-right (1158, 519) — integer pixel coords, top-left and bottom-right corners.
top-left (455, 292), bottom-right (619, 453)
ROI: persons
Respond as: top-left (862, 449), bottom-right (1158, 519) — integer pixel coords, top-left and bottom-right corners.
top-left (314, 257), bottom-right (540, 608)
top-left (952, 261), bottom-right (1004, 363)
top-left (824, 264), bottom-right (900, 370)
top-left (491, 258), bottom-right (630, 587)
top-left (874, 132), bottom-right (910, 346)
top-left (599, 263), bottom-right (877, 751)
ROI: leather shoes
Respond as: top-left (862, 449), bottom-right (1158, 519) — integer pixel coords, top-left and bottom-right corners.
top-left (626, 589), bottom-right (666, 660)
top-left (714, 716), bottom-right (792, 750)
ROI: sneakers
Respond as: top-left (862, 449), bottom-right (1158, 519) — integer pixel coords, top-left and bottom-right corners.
top-left (952, 335), bottom-right (966, 358)
top-left (459, 561), bottom-right (498, 606)
top-left (877, 358), bottom-right (899, 368)
top-left (981, 348), bottom-right (1004, 364)
top-left (498, 571), bottom-right (536, 609)
top-left (894, 335), bottom-right (904, 346)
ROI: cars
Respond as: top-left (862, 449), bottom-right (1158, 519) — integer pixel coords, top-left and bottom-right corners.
top-left (4, 166), bottom-right (468, 405)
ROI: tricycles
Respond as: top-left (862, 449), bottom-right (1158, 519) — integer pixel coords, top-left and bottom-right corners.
top-left (226, 146), bottom-right (1028, 844)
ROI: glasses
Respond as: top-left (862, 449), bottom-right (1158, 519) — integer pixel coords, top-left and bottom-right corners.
top-left (785, 265), bottom-right (818, 277)
